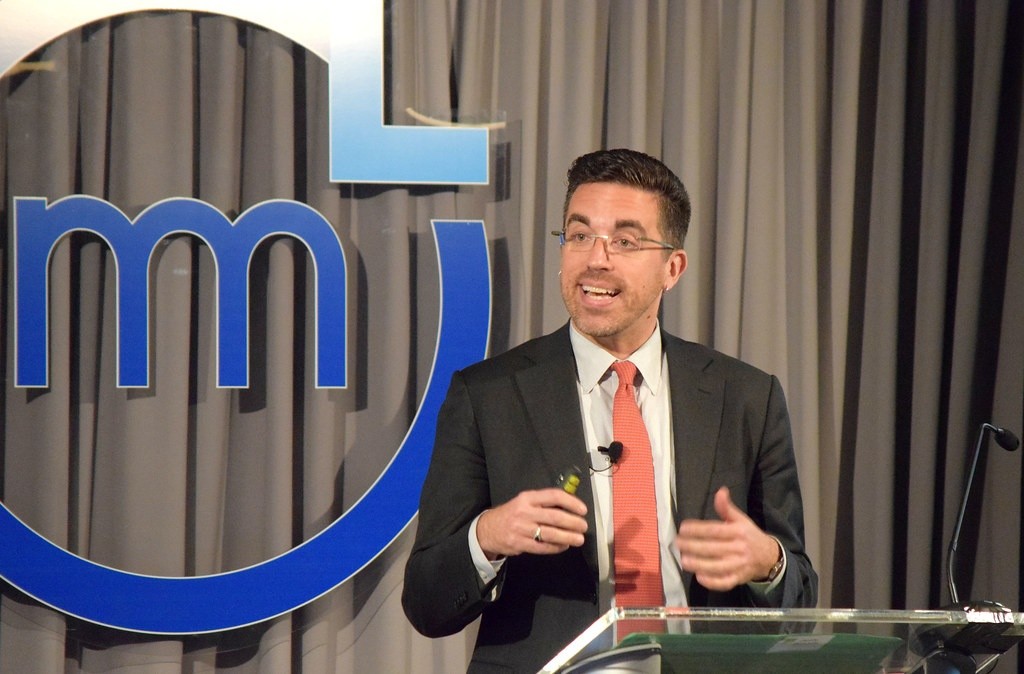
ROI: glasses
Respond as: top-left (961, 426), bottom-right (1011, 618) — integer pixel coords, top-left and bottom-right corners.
top-left (552, 227), bottom-right (674, 258)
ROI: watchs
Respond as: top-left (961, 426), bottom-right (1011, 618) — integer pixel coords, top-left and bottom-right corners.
top-left (768, 557), bottom-right (783, 582)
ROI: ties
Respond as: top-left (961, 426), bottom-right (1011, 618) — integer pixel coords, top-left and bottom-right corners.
top-left (609, 361), bottom-right (666, 646)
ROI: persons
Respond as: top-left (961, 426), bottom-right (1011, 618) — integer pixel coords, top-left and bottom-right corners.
top-left (401, 149), bottom-right (818, 674)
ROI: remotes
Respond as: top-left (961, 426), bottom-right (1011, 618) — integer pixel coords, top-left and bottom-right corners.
top-left (551, 463), bottom-right (583, 511)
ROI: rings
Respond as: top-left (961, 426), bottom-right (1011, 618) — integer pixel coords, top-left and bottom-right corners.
top-left (533, 523), bottom-right (540, 542)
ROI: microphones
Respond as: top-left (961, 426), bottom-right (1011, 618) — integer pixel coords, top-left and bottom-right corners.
top-left (607, 442), bottom-right (623, 464)
top-left (909, 423), bottom-right (1020, 658)
